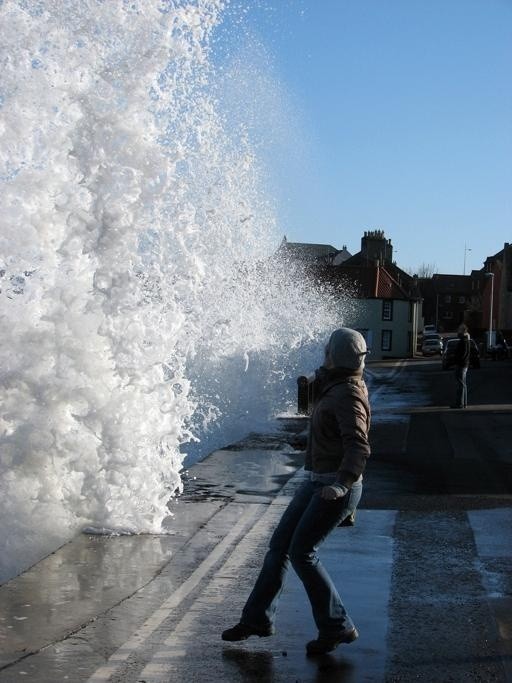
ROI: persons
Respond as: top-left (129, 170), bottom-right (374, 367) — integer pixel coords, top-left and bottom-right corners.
top-left (451, 323), bottom-right (472, 409)
top-left (221, 327), bottom-right (372, 656)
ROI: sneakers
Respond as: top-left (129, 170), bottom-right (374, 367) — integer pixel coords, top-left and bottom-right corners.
top-left (222, 624), bottom-right (273, 641)
top-left (307, 628), bottom-right (358, 655)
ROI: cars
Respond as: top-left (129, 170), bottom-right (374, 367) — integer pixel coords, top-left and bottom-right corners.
top-left (422, 335), bottom-right (443, 355)
top-left (423, 325), bottom-right (437, 334)
top-left (443, 338), bottom-right (481, 369)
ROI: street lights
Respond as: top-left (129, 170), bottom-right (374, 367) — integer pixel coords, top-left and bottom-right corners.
top-left (486, 273), bottom-right (495, 348)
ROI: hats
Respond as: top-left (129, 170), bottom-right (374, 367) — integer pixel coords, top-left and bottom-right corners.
top-left (331, 328), bottom-right (369, 370)
